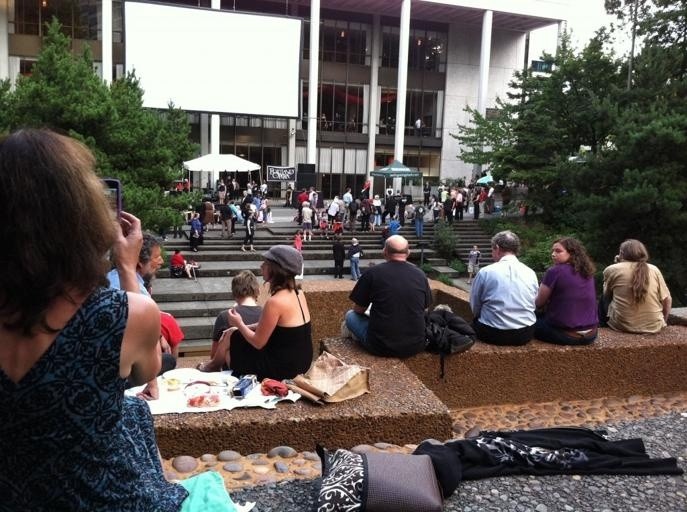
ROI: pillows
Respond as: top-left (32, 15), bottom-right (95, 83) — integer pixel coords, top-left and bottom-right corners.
top-left (315, 440), bottom-right (445, 512)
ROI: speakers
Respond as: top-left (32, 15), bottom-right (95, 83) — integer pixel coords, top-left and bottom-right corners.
top-left (299, 163), bottom-right (315, 172)
top-left (298, 171), bottom-right (316, 190)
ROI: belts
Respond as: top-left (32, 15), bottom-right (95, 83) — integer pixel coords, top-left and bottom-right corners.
top-left (563, 330), bottom-right (593, 338)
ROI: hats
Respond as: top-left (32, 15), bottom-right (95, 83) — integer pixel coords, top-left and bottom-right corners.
top-left (262, 245), bottom-right (302, 275)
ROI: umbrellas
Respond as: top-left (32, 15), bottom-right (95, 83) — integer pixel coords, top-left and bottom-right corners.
top-left (181, 152), bottom-right (261, 205)
top-left (476, 175), bottom-right (504, 184)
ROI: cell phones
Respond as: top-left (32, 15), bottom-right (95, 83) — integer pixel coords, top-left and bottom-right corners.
top-left (99, 178), bottom-right (121, 224)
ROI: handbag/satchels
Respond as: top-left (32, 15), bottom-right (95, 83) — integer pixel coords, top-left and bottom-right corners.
top-left (425, 304), bottom-right (476, 355)
top-left (286, 351), bottom-right (371, 406)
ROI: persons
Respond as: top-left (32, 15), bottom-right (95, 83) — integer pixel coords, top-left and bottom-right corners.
top-left (100, 232), bottom-right (178, 393)
top-left (209, 269), bottom-right (264, 371)
top-left (535, 236), bottom-right (600, 346)
top-left (341, 234), bottom-right (434, 358)
top-left (298, 109), bottom-right (426, 136)
top-left (140, 273), bottom-right (184, 361)
top-left (195, 246), bottom-right (313, 384)
top-left (602, 239), bottom-right (673, 336)
top-left (0, 128), bottom-right (191, 512)
top-left (470, 230), bottom-right (540, 347)
top-left (162, 176), bottom-right (529, 284)
